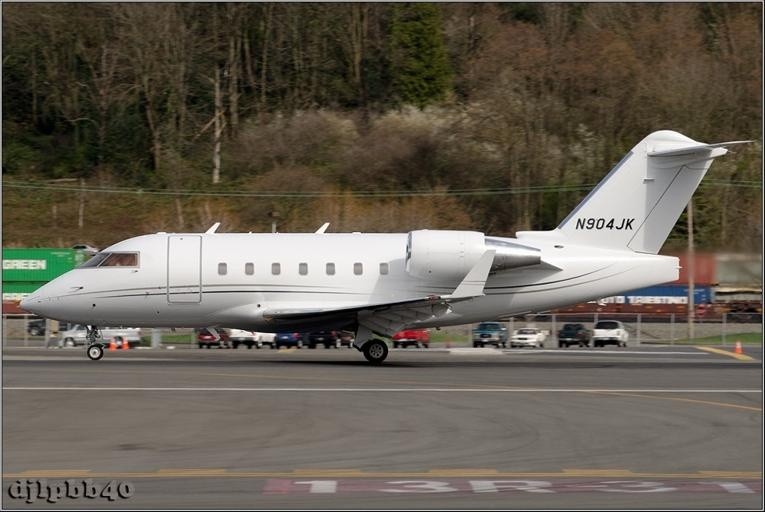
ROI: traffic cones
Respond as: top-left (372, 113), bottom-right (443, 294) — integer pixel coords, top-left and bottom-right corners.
top-left (109, 337), bottom-right (117, 350)
top-left (736, 339), bottom-right (743, 354)
top-left (123, 335), bottom-right (129, 350)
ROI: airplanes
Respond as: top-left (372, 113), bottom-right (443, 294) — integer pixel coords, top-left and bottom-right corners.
top-left (18, 130), bottom-right (759, 364)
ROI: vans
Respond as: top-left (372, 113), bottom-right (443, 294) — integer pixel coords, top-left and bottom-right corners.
top-left (59, 324), bottom-right (141, 349)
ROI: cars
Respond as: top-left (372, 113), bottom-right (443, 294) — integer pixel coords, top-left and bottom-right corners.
top-left (511, 328), bottom-right (546, 349)
top-left (557, 324), bottom-right (591, 347)
top-left (393, 329), bottom-right (431, 347)
top-left (197, 328), bottom-right (353, 349)
top-left (472, 322), bottom-right (508, 348)
top-left (592, 320), bottom-right (629, 347)
